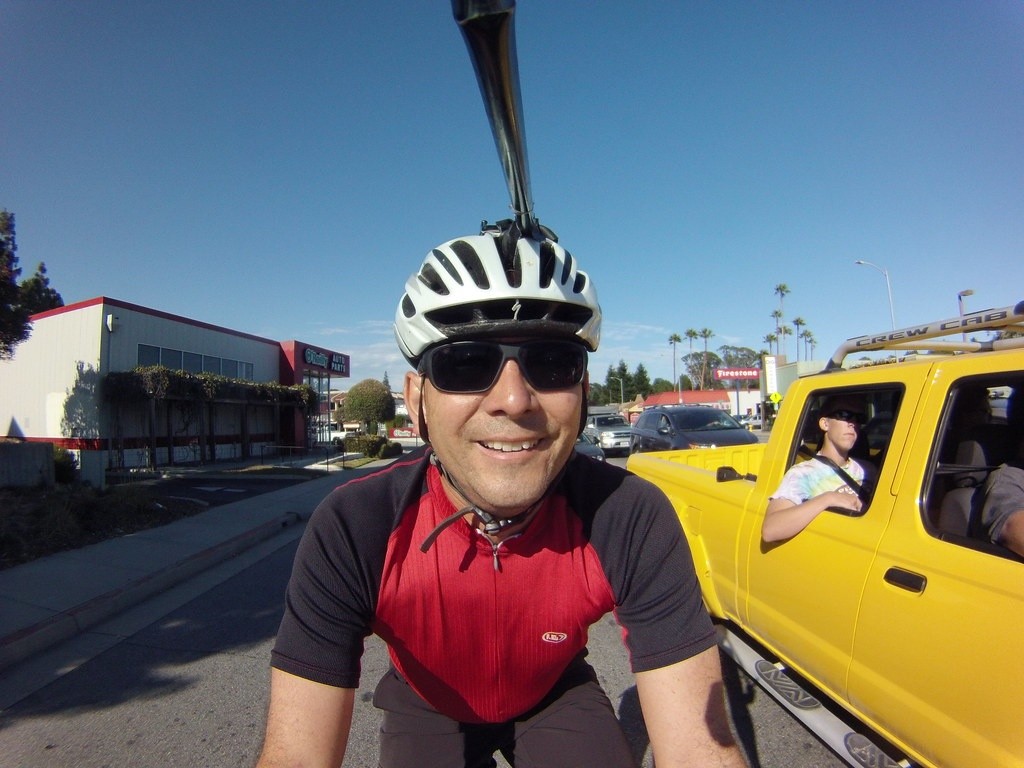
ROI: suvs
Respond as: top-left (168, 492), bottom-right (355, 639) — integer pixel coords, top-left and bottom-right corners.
top-left (628, 403), bottom-right (759, 454)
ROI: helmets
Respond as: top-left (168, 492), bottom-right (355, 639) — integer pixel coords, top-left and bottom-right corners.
top-left (394, 230), bottom-right (603, 374)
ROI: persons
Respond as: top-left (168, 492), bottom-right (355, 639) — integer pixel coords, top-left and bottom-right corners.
top-left (967, 378), bottom-right (1024, 559)
top-left (762, 388), bottom-right (877, 544)
top-left (254, 227), bottom-right (750, 768)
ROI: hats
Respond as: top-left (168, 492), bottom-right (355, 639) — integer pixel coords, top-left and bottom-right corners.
top-left (817, 394), bottom-right (869, 421)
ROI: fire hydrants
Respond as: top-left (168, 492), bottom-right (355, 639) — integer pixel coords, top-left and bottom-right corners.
top-left (748, 425), bottom-right (753, 431)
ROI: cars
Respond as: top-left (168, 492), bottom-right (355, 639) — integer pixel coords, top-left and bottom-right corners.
top-left (740, 413), bottom-right (761, 430)
top-left (723, 413), bottom-right (747, 426)
top-left (575, 432), bottom-right (605, 462)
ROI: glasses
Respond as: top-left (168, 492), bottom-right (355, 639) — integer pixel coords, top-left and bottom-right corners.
top-left (833, 409), bottom-right (865, 425)
top-left (416, 335), bottom-right (589, 395)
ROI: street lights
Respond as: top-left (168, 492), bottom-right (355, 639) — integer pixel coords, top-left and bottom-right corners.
top-left (957, 288), bottom-right (974, 342)
top-left (855, 260), bottom-right (899, 362)
top-left (611, 377), bottom-right (623, 406)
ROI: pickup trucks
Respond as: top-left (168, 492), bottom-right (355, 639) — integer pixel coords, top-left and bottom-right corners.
top-left (624, 303), bottom-right (1024, 768)
top-left (585, 414), bottom-right (632, 450)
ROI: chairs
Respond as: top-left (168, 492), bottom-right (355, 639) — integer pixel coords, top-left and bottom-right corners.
top-left (815, 429), bottom-right (870, 459)
top-left (939, 423), bottom-right (1017, 538)
